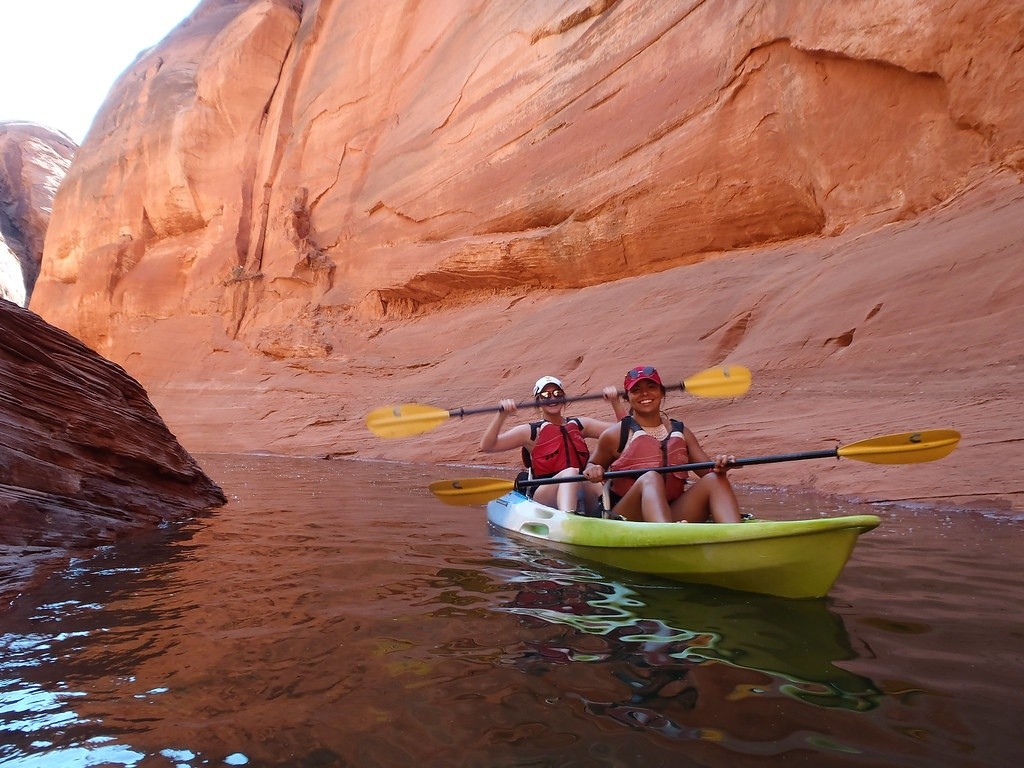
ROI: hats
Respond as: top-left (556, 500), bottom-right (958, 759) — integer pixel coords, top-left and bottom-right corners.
top-left (624, 366), bottom-right (664, 393)
top-left (533, 375), bottom-right (565, 397)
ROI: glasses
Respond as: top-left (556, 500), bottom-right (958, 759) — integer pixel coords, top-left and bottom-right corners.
top-left (540, 389), bottom-right (564, 399)
top-left (627, 367), bottom-right (656, 379)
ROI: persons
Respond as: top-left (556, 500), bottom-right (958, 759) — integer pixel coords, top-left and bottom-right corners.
top-left (480, 375), bottom-right (629, 513)
top-left (583, 366), bottom-right (742, 523)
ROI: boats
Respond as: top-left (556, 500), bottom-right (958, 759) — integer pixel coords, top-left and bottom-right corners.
top-left (484, 483), bottom-right (883, 600)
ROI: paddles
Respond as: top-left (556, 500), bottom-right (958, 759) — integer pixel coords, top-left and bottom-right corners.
top-left (364, 364), bottom-right (752, 441)
top-left (428, 428), bottom-right (963, 508)
